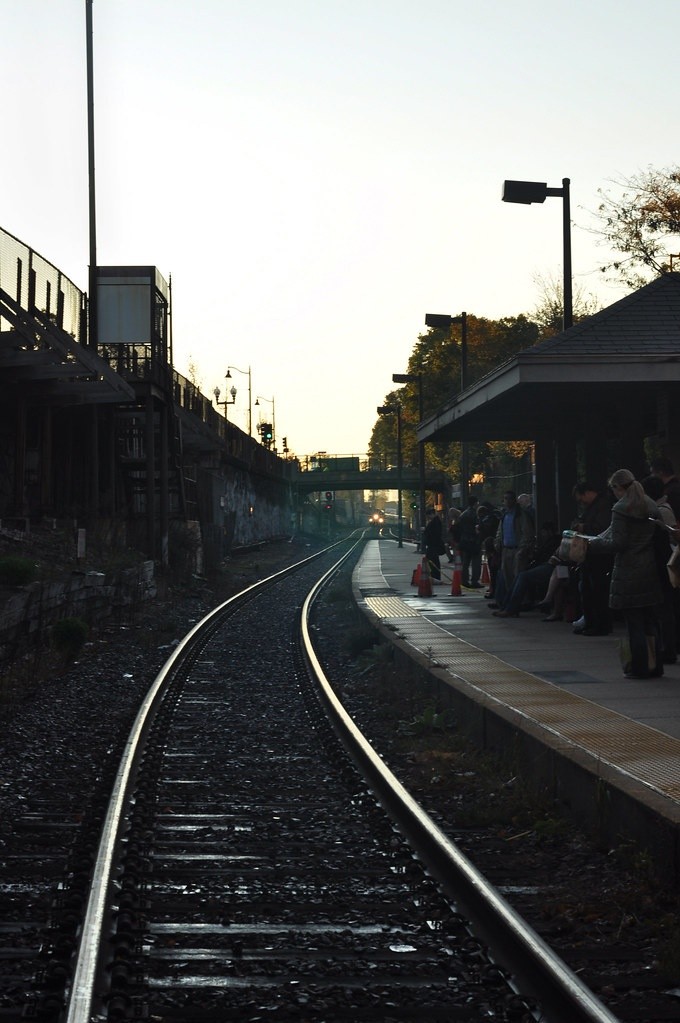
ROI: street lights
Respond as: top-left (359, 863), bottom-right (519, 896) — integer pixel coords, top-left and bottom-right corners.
top-left (426, 312), bottom-right (466, 511)
top-left (225, 365), bottom-right (251, 437)
top-left (377, 405), bottom-right (403, 548)
top-left (255, 395), bottom-right (275, 453)
top-left (214, 386), bottom-right (237, 421)
top-left (501, 178), bottom-right (573, 333)
top-left (392, 374), bottom-right (426, 554)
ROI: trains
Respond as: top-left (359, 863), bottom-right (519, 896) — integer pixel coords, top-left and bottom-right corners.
top-left (370, 509), bottom-right (412, 528)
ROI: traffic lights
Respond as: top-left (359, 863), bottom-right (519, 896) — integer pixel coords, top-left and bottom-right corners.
top-left (267, 424), bottom-right (272, 441)
top-left (326, 505), bottom-right (331, 511)
top-left (283, 438), bottom-right (287, 448)
top-left (326, 492), bottom-right (332, 501)
top-left (261, 423), bottom-right (267, 436)
top-left (412, 491), bottom-right (419, 497)
top-left (411, 502), bottom-right (420, 510)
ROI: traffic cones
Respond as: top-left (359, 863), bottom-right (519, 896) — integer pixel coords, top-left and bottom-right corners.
top-left (411, 563), bottom-right (422, 588)
top-left (454, 550), bottom-right (465, 574)
top-left (413, 557), bottom-right (437, 597)
top-left (481, 563), bottom-right (490, 583)
top-left (447, 570), bottom-right (466, 596)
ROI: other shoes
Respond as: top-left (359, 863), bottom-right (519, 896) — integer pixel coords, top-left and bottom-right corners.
top-left (624, 672), bottom-right (645, 679)
top-left (535, 599), bottom-right (554, 607)
top-left (491, 607), bottom-right (519, 617)
top-left (471, 582), bottom-right (484, 587)
top-left (573, 615), bottom-right (584, 626)
top-left (461, 580), bottom-right (470, 587)
top-left (487, 601), bottom-right (504, 608)
top-left (541, 614), bottom-right (561, 620)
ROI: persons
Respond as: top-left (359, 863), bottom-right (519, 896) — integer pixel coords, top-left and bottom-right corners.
top-left (421, 508), bottom-right (446, 580)
top-left (448, 459), bottom-right (680, 679)
